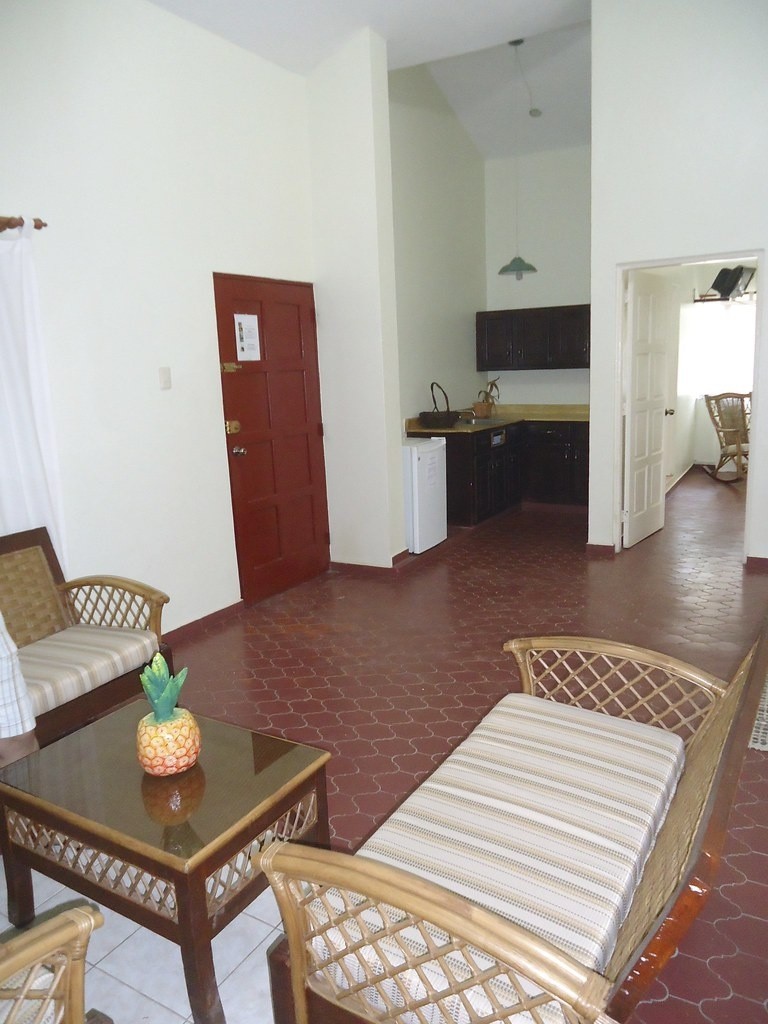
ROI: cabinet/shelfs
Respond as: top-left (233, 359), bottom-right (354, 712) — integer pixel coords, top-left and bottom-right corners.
top-left (407, 420), bottom-right (589, 527)
top-left (476, 304), bottom-right (591, 373)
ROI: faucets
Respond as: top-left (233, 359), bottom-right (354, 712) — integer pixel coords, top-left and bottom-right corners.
top-left (456, 408), bottom-right (478, 417)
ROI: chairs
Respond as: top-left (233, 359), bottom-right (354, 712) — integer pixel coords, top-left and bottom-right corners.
top-left (700, 391), bottom-right (753, 484)
top-left (0, 526), bottom-right (179, 749)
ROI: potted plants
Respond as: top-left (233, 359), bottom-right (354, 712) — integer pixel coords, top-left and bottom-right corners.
top-left (470, 376), bottom-right (501, 420)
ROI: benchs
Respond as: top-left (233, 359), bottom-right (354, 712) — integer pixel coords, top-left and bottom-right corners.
top-left (260, 634), bottom-right (761, 1024)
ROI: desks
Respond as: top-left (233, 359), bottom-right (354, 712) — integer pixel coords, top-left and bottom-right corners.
top-left (0, 689), bottom-right (335, 1024)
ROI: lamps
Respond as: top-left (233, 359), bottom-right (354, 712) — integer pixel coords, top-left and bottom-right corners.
top-left (499, 257), bottom-right (539, 281)
top-left (693, 265), bottom-right (757, 303)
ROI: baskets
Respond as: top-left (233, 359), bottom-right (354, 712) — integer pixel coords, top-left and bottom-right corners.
top-left (419, 382), bottom-right (461, 428)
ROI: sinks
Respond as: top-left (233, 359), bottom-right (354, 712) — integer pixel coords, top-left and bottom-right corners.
top-left (460, 417), bottom-right (507, 427)
